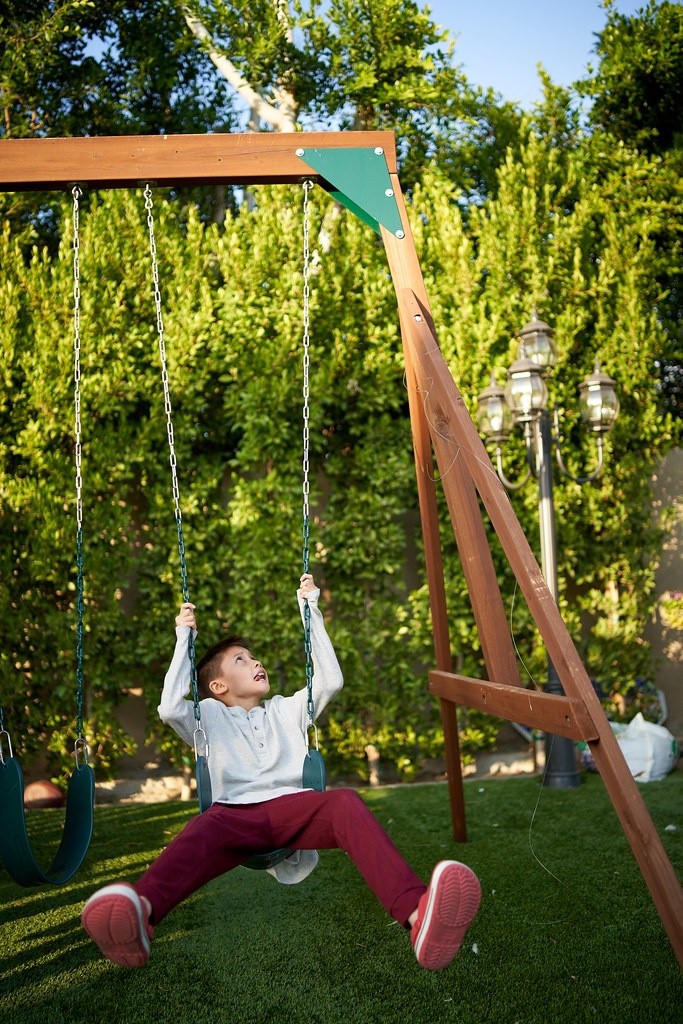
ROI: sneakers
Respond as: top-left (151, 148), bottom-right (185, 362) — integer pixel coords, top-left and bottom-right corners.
top-left (81, 882), bottom-right (153, 969)
top-left (410, 860), bottom-right (482, 970)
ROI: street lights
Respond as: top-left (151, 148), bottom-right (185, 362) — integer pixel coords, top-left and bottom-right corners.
top-left (476, 315), bottom-right (621, 788)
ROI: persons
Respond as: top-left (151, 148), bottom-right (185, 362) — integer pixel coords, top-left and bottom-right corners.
top-left (82, 573), bottom-right (481, 971)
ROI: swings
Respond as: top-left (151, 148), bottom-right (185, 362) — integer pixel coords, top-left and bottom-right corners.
top-left (2, 186), bottom-right (95, 889)
top-left (142, 178), bottom-right (328, 872)
